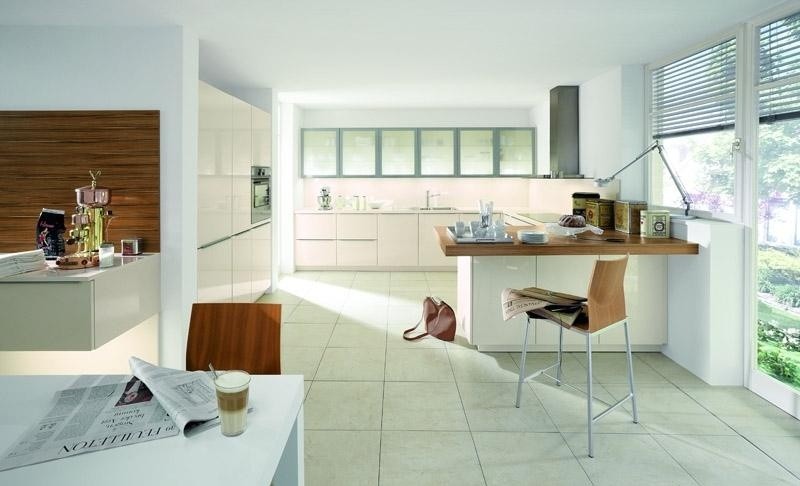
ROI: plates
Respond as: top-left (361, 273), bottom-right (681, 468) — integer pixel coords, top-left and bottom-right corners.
top-left (516, 229), bottom-right (549, 244)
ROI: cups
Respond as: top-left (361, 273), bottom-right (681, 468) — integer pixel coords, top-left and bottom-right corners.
top-left (469, 221), bottom-right (480, 236)
top-left (213, 368), bottom-right (251, 437)
top-left (455, 222), bottom-right (465, 238)
top-left (478, 200), bottom-right (493, 232)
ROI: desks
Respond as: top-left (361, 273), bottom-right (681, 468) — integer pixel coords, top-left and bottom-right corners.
top-left (1, 372), bottom-right (306, 486)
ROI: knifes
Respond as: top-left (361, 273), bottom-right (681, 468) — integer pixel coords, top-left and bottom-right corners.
top-left (579, 236), bottom-right (626, 243)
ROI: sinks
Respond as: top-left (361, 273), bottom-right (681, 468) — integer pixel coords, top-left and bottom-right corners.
top-left (419, 206), bottom-right (458, 211)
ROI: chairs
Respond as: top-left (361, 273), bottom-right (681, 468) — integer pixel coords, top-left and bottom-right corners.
top-left (184, 302), bottom-right (283, 377)
top-left (510, 250), bottom-right (640, 462)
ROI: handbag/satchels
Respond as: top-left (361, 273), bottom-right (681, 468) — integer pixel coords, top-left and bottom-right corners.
top-left (421, 294), bottom-right (457, 343)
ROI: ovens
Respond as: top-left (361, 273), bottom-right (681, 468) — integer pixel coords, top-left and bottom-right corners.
top-left (251, 166), bottom-right (273, 224)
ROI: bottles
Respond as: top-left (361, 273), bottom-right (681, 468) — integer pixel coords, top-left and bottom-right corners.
top-left (316, 188), bottom-right (332, 210)
top-left (336, 194), bottom-right (367, 210)
top-left (99, 243), bottom-right (115, 267)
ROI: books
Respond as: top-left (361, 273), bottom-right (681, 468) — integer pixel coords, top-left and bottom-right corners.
top-left (0, 249), bottom-right (47, 279)
top-left (501, 286), bottom-right (588, 329)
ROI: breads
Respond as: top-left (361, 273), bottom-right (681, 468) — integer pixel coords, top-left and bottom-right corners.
top-left (558, 214), bottom-right (587, 227)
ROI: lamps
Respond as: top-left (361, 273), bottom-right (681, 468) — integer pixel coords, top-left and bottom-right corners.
top-left (592, 138), bottom-right (697, 221)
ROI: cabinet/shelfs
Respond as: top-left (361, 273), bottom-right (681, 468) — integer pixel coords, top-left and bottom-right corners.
top-left (197, 237), bottom-right (233, 308)
top-left (253, 222), bottom-right (272, 301)
top-left (232, 229), bottom-right (253, 306)
top-left (301, 127), bottom-right (380, 177)
top-left (536, 253), bottom-right (671, 355)
top-left (0, 250), bottom-right (161, 353)
top-left (198, 79), bottom-right (232, 248)
top-left (454, 253), bottom-right (536, 354)
top-left (380, 128), bottom-right (458, 177)
top-left (232, 96), bottom-right (252, 237)
top-left (377, 213), bottom-right (459, 272)
top-left (459, 213), bottom-right (502, 223)
top-left (252, 105), bottom-right (272, 167)
top-left (458, 128), bottom-right (536, 177)
top-left (292, 213), bottom-right (377, 271)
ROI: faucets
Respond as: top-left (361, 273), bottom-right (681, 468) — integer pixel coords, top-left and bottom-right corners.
top-left (426, 190), bottom-right (440, 207)
